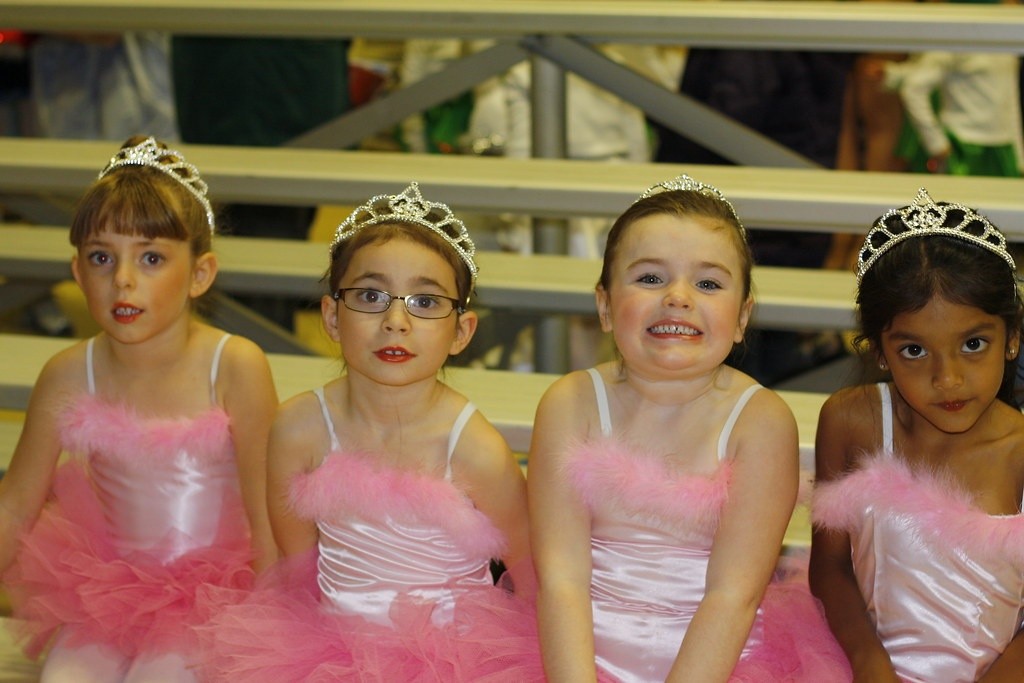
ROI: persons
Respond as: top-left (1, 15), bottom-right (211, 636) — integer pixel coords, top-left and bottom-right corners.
top-left (189, 180), bottom-right (539, 683)
top-left (0, 137), bottom-right (281, 683)
top-left (527, 174), bottom-right (801, 682)
top-left (18, 26), bottom-right (1024, 394)
top-left (773, 187), bottom-right (1024, 683)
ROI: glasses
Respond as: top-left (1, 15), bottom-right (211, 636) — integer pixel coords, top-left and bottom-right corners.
top-left (325, 278), bottom-right (468, 321)
